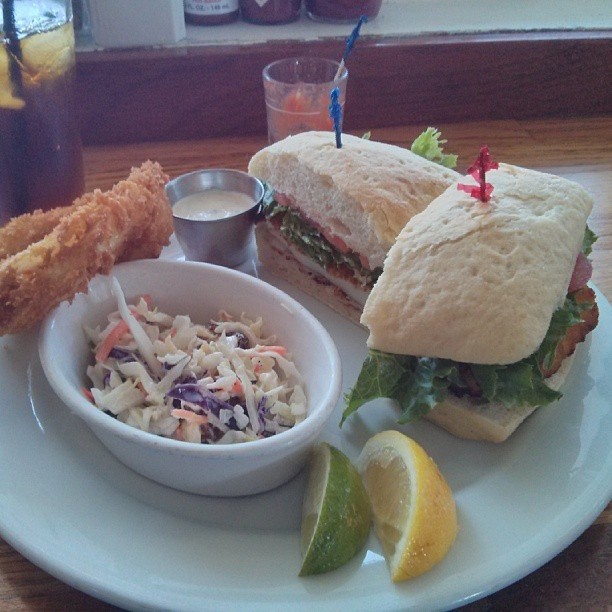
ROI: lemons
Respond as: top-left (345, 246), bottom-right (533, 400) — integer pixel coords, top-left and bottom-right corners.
top-left (354, 429), bottom-right (458, 582)
top-left (296, 442), bottom-right (371, 578)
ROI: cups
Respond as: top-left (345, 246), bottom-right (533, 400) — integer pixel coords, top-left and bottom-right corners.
top-left (0, 0), bottom-right (86, 228)
top-left (261, 56), bottom-right (349, 146)
top-left (163, 168), bottom-right (265, 270)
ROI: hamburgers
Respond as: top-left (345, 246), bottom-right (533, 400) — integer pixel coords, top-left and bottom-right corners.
top-left (246, 130), bottom-right (466, 332)
top-left (340, 160), bottom-right (597, 442)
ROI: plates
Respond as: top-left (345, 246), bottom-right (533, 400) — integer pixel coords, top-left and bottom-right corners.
top-left (0, 218), bottom-right (610, 612)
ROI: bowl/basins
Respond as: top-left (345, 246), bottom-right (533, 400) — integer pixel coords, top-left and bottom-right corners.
top-left (36, 258), bottom-right (342, 498)
